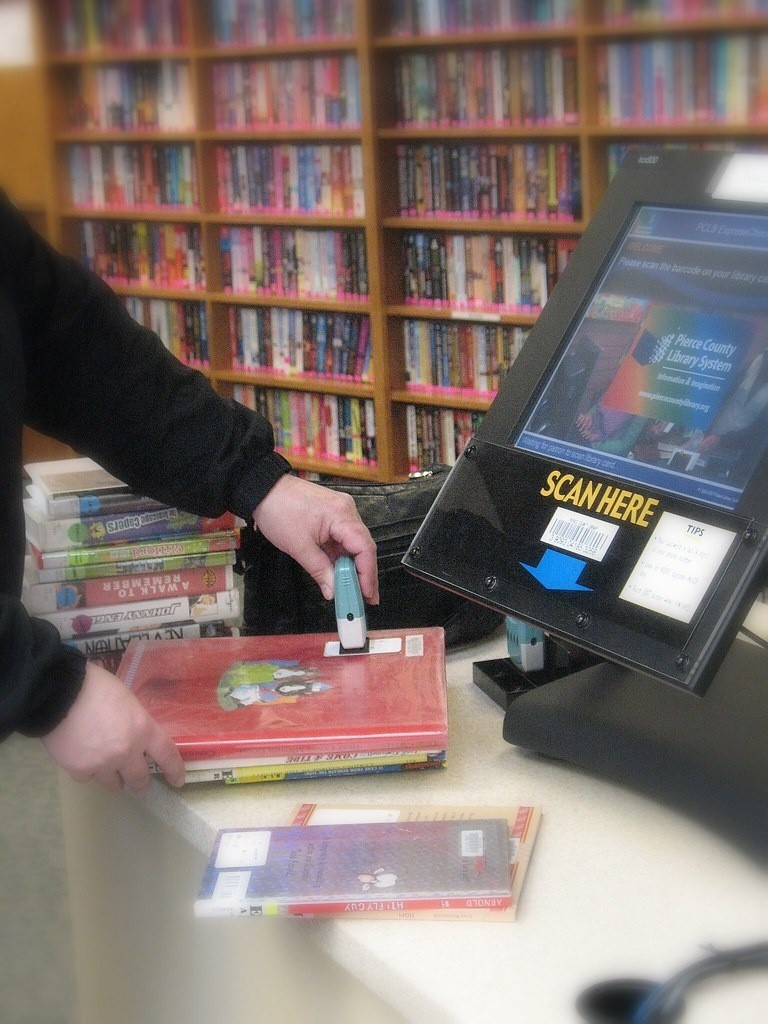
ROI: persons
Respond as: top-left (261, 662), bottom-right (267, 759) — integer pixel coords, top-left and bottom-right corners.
top-left (0, 188), bottom-right (381, 797)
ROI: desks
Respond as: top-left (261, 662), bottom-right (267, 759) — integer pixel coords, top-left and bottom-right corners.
top-left (0, 594), bottom-right (768, 1024)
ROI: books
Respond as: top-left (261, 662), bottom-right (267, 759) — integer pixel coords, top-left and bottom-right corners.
top-left (22, 458), bottom-right (452, 785)
top-left (52, 0), bottom-right (768, 487)
top-left (195, 797), bottom-right (543, 924)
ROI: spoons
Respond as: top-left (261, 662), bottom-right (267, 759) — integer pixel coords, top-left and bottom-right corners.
top-left (578, 941), bottom-right (768, 1024)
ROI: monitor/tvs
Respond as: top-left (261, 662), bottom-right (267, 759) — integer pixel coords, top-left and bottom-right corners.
top-left (515, 205), bottom-right (768, 512)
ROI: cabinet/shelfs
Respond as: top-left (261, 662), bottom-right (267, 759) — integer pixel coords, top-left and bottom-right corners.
top-left (30, 0), bottom-right (768, 486)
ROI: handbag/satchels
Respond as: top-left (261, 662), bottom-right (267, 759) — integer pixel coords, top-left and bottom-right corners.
top-left (241, 467), bottom-right (504, 650)
